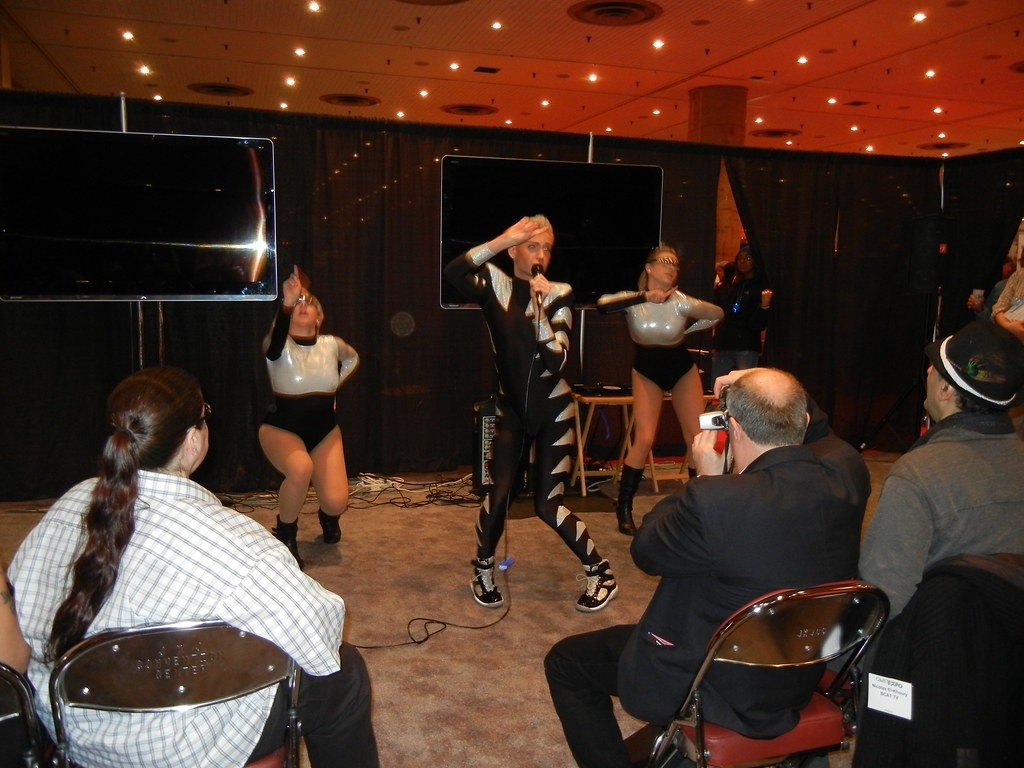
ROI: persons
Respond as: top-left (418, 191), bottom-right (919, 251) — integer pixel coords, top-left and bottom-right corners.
top-left (445, 215), bottom-right (619, 612)
top-left (711, 245), bottom-right (773, 392)
top-left (806, 319), bottom-right (1024, 768)
top-left (597, 246), bottom-right (724, 535)
top-left (543, 367), bottom-right (871, 768)
top-left (0, 365), bottom-right (381, 768)
top-left (258, 265), bottom-right (359, 570)
top-left (967, 216), bottom-right (1024, 342)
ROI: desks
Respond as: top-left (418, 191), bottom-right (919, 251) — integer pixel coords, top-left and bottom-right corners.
top-left (563, 391), bottom-right (727, 497)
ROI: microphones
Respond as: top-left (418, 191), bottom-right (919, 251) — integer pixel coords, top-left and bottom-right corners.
top-left (530, 263), bottom-right (544, 306)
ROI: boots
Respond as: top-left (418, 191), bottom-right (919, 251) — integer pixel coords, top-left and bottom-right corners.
top-left (271, 513), bottom-right (300, 569)
top-left (615, 463), bottom-right (645, 535)
top-left (576, 559), bottom-right (618, 612)
top-left (470, 556), bottom-right (505, 607)
top-left (317, 506), bottom-right (344, 544)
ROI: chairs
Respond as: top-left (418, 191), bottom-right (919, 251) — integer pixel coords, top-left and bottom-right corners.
top-left (0, 663), bottom-right (54, 768)
top-left (848, 553), bottom-right (1024, 768)
top-left (651, 578), bottom-right (890, 768)
top-left (49, 617), bottom-right (314, 768)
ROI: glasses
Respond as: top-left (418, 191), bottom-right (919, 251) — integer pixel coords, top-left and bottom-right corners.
top-left (649, 256), bottom-right (679, 268)
top-left (737, 256), bottom-right (752, 261)
top-left (186, 403), bottom-right (212, 432)
top-left (296, 295), bottom-right (321, 310)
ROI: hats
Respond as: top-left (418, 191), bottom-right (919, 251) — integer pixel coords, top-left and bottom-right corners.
top-left (736, 246), bottom-right (758, 265)
top-left (924, 319), bottom-right (1024, 409)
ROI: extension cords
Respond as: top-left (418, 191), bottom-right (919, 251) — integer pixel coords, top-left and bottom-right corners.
top-left (362, 482), bottom-right (400, 493)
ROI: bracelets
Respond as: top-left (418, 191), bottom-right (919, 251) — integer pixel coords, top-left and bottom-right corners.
top-left (762, 306), bottom-right (769, 309)
top-left (733, 280), bottom-right (745, 313)
top-left (990, 309), bottom-right (1002, 324)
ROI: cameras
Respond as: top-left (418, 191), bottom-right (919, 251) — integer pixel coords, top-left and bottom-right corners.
top-left (698, 384), bottom-right (731, 432)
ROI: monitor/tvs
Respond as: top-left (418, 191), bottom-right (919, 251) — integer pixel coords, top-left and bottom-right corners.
top-left (439, 155), bottom-right (664, 310)
top-left (0, 126), bottom-right (279, 303)
